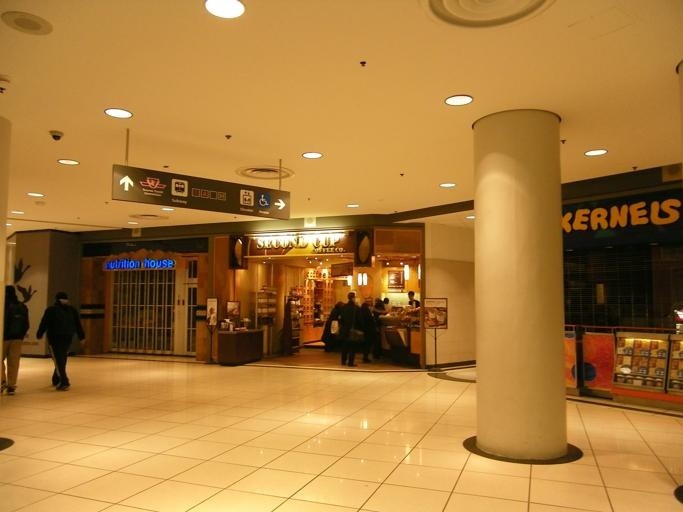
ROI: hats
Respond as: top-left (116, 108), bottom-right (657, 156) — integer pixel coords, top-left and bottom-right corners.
top-left (55, 292), bottom-right (68, 299)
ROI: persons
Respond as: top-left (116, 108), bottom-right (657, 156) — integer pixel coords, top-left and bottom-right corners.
top-left (36, 291), bottom-right (87, 390)
top-left (1, 285), bottom-right (30, 396)
top-left (408, 291), bottom-right (420, 307)
top-left (323, 292), bottom-right (391, 366)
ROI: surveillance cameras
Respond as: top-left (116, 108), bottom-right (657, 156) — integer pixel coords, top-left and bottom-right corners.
top-left (50, 131), bottom-right (63, 140)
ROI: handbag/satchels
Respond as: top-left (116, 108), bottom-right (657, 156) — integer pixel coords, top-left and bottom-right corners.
top-left (347, 329), bottom-right (364, 343)
top-left (330, 320), bottom-right (340, 337)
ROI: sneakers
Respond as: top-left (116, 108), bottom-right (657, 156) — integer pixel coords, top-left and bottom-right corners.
top-left (0, 381), bottom-right (69, 397)
